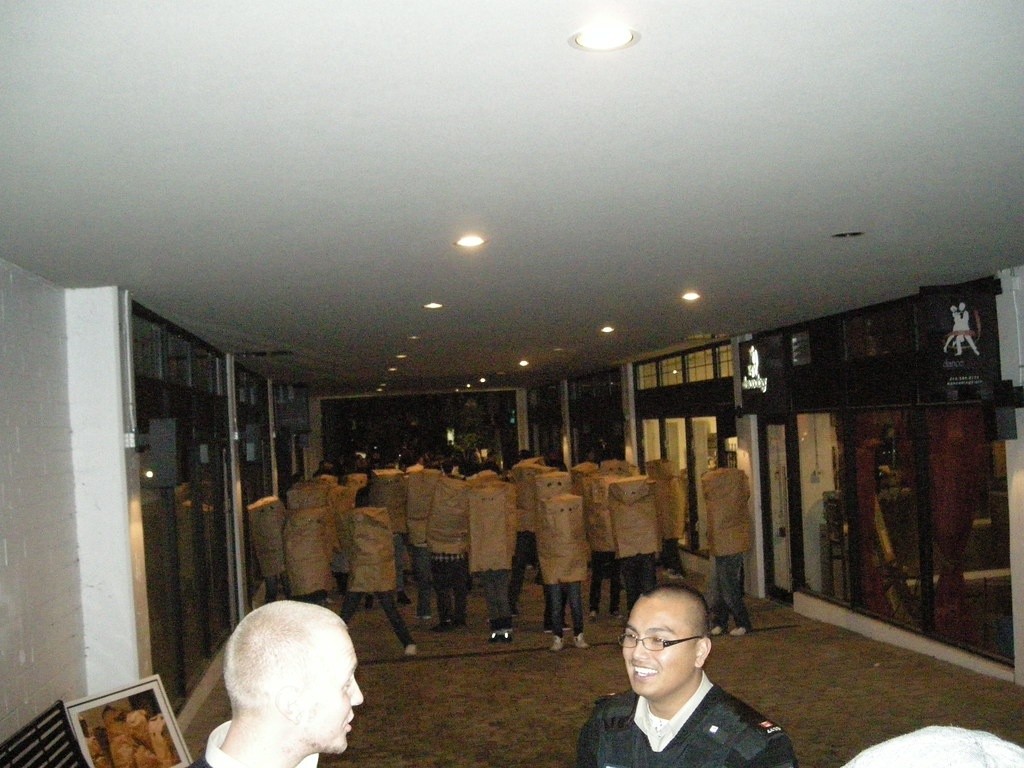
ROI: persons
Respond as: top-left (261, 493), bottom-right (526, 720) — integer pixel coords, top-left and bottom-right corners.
top-left (247, 445), bottom-right (754, 656)
top-left (574, 582), bottom-right (798, 768)
top-left (186, 600), bottom-right (364, 768)
top-left (839, 725), bottom-right (1024, 768)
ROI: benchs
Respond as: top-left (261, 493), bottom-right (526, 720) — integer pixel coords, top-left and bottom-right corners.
top-left (0, 700), bottom-right (90, 768)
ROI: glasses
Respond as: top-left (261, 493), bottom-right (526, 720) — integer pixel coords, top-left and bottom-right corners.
top-left (616, 633), bottom-right (703, 652)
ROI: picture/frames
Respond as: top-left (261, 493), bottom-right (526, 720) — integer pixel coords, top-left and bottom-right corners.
top-left (63, 674), bottom-right (194, 768)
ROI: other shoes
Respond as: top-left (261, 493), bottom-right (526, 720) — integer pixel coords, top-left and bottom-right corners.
top-left (562, 623), bottom-right (571, 630)
top-left (588, 611), bottom-right (597, 624)
top-left (729, 628), bottom-right (748, 636)
top-left (609, 609), bottom-right (625, 619)
top-left (365, 594), bottom-right (374, 610)
top-left (544, 627), bottom-right (553, 634)
top-left (404, 643), bottom-right (417, 657)
top-left (487, 633), bottom-right (513, 646)
top-left (549, 635), bottom-right (565, 653)
top-left (708, 626), bottom-right (727, 636)
top-left (509, 603), bottom-right (520, 619)
top-left (415, 612), bottom-right (431, 620)
top-left (574, 633), bottom-right (591, 650)
top-left (430, 618), bottom-right (468, 634)
top-left (395, 589), bottom-right (411, 604)
top-left (662, 567), bottom-right (686, 579)
